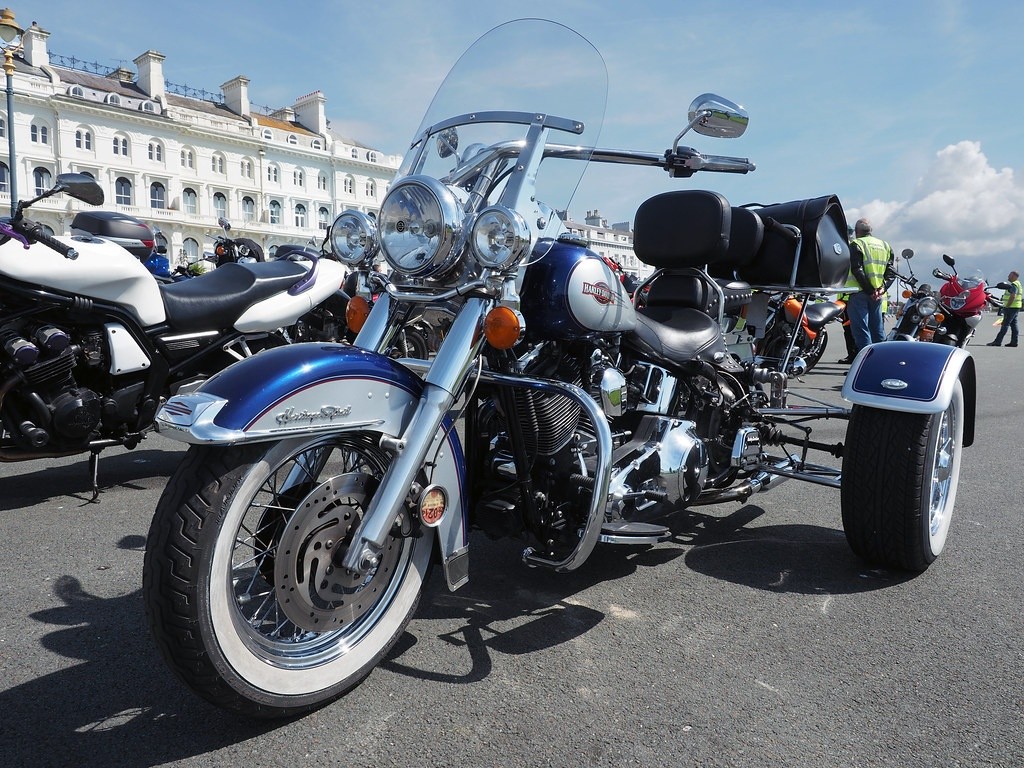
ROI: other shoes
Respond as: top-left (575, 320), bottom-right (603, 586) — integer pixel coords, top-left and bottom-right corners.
top-left (1005, 342), bottom-right (1019, 347)
top-left (838, 356), bottom-right (855, 364)
top-left (987, 340), bottom-right (1001, 347)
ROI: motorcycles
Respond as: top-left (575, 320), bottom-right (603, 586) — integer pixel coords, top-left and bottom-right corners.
top-left (609, 257), bottom-right (651, 307)
top-left (0, 173), bottom-right (356, 505)
top-left (143, 214), bottom-right (450, 377)
top-left (140, 17), bottom-right (978, 724)
top-left (756, 291), bottom-right (846, 383)
top-left (887, 249), bottom-right (970, 342)
top-left (933, 254), bottom-right (1012, 350)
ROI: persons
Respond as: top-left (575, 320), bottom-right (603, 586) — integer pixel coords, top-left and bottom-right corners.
top-left (843, 218), bottom-right (894, 376)
top-left (837, 226), bottom-right (863, 364)
top-left (987, 271), bottom-right (1023, 347)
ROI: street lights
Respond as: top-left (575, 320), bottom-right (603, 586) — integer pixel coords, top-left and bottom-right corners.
top-left (895, 257), bottom-right (900, 305)
top-left (0, 6), bottom-right (25, 217)
